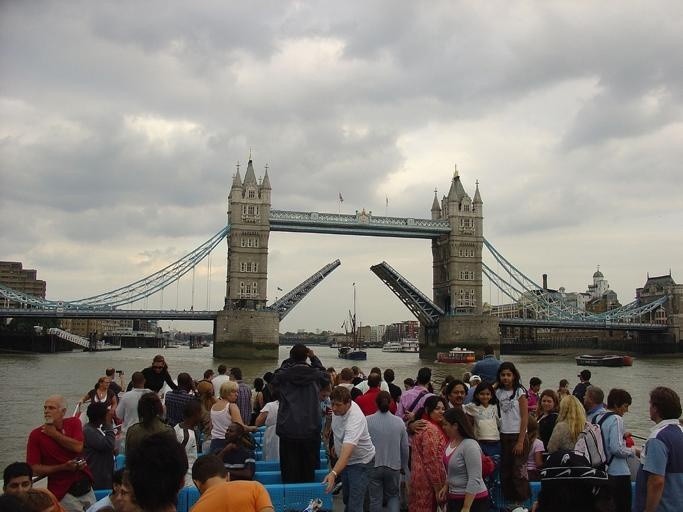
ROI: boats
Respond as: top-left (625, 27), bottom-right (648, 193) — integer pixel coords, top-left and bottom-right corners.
top-left (338, 346), bottom-right (367, 361)
top-left (383, 338), bottom-right (419, 352)
top-left (436, 347), bottom-right (476, 363)
top-left (576, 353), bottom-right (634, 366)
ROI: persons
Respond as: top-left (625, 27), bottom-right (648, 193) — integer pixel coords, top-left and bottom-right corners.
top-left (269, 344), bottom-right (330, 483)
top-left (125, 432), bottom-right (189, 512)
top-left (513, 415), bottom-right (544, 483)
top-left (442, 380), bottom-right (467, 416)
top-left (72, 376), bottom-right (121, 426)
top-left (87, 468), bottom-right (128, 512)
top-left (355, 368), bottom-right (390, 394)
top-left (493, 362), bottom-right (531, 501)
top-left (186, 454), bottom-right (274, 512)
top-left (384, 369), bottom-right (402, 402)
top-left (584, 384), bottom-right (606, 424)
top-left (127, 354), bottom-right (177, 391)
top-left (590, 388), bottom-right (641, 512)
top-left (408, 396), bottom-right (447, 512)
top-left (115, 371), bottom-right (161, 429)
top-left (535, 389), bottom-right (560, 450)
top-left (217, 421), bottom-right (254, 463)
top-left (250, 378), bottom-right (263, 426)
top-left (365, 391), bottom-right (409, 512)
top-left (338, 369), bottom-right (354, 392)
top-left (229, 368), bottom-right (252, 424)
top-left (440, 408), bottom-right (491, 512)
top-left (321, 386), bottom-right (376, 512)
top-left (117, 468), bottom-right (141, 512)
top-left (464, 382), bottom-right (501, 455)
top-left (327, 368), bottom-right (337, 386)
top-left (26, 394), bottom-right (96, 512)
top-left (0, 461), bottom-right (67, 512)
top-left (210, 382), bottom-right (258, 456)
top-left (105, 367), bottom-right (125, 406)
top-left (395, 367), bottom-right (438, 425)
top-left (557, 379), bottom-right (572, 403)
top-left (200, 369), bottom-right (214, 383)
top-left (355, 373), bottom-right (397, 416)
top-left (214, 365), bottom-right (230, 398)
top-left (403, 378), bottom-right (415, 391)
top-left (408, 379), bottom-right (482, 434)
top-left (80, 402), bottom-right (122, 489)
top-left (471, 345), bottom-right (504, 386)
top-left (320, 385), bottom-right (332, 438)
top-left (636, 386), bottom-right (683, 512)
top-left (527, 377), bottom-right (541, 412)
top-left (197, 381), bottom-right (217, 431)
top-left (548, 395), bottom-right (587, 451)
top-left (165, 373), bottom-right (198, 425)
top-left (352, 366), bottom-right (366, 380)
top-left (574, 370), bottom-right (592, 403)
top-left (125, 392), bottom-right (178, 453)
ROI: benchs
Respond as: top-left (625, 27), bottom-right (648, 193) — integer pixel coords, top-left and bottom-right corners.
top-left (92, 483), bottom-right (336, 512)
top-left (255, 458), bottom-right (328, 472)
top-left (253, 469), bottom-right (331, 484)
top-left (255, 449), bottom-right (327, 460)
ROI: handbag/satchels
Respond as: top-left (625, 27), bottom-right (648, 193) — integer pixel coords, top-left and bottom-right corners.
top-left (228, 404), bottom-right (262, 452)
top-left (67, 476), bottom-right (92, 499)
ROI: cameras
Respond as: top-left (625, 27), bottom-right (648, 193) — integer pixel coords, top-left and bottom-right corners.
top-left (116, 371), bottom-right (122, 374)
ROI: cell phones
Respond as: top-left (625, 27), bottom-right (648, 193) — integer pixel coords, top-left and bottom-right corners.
top-left (75, 459), bottom-right (83, 464)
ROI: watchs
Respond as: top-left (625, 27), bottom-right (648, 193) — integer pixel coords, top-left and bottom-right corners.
top-left (331, 470), bottom-right (337, 476)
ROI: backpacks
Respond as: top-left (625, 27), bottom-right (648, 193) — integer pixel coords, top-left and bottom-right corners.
top-left (592, 412), bottom-right (622, 471)
top-left (575, 422), bottom-right (606, 465)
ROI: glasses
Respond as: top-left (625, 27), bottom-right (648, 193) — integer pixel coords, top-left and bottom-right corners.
top-left (118, 489), bottom-right (129, 498)
top-left (152, 365), bottom-right (164, 370)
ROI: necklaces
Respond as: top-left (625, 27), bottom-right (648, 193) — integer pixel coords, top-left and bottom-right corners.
top-left (99, 389), bottom-right (106, 397)
